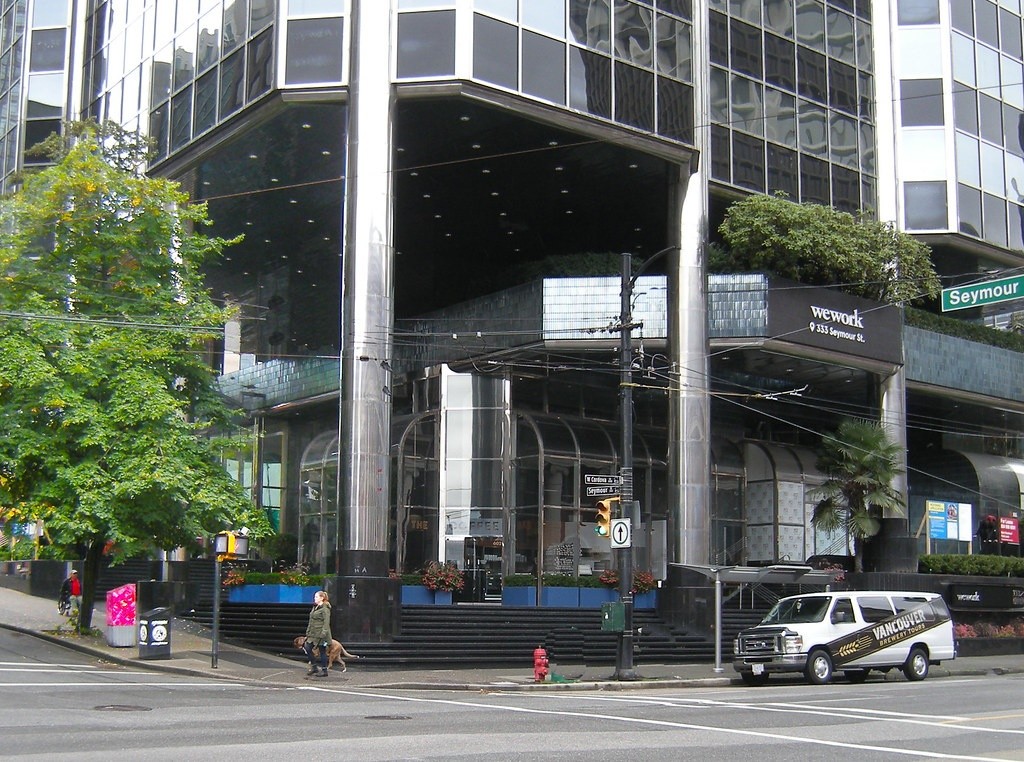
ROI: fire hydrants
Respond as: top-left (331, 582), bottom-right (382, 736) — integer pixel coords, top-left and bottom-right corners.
top-left (534, 645), bottom-right (551, 684)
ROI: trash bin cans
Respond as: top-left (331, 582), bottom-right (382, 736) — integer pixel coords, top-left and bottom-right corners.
top-left (138, 606), bottom-right (172, 660)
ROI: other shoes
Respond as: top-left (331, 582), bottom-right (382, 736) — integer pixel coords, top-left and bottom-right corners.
top-left (307, 665), bottom-right (319, 675)
top-left (315, 667), bottom-right (328, 677)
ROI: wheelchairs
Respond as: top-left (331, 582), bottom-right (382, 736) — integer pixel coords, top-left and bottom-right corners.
top-left (58, 594), bottom-right (80, 615)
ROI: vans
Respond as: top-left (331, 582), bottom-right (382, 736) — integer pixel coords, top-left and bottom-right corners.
top-left (733, 590), bottom-right (960, 684)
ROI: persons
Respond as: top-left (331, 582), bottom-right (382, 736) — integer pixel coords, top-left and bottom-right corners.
top-left (303, 590), bottom-right (332, 677)
top-left (61, 570), bottom-right (82, 616)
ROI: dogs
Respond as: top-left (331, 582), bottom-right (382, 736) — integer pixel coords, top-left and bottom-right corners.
top-left (292, 637), bottom-right (359, 673)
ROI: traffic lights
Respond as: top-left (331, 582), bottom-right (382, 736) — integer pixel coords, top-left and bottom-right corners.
top-left (213, 529), bottom-right (250, 557)
top-left (596, 499), bottom-right (612, 541)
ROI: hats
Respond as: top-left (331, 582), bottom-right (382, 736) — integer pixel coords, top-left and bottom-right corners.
top-left (70, 570), bottom-right (78, 574)
